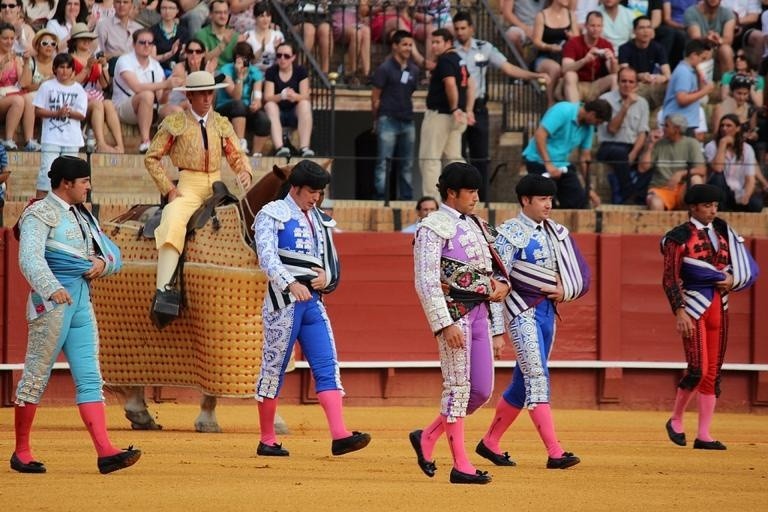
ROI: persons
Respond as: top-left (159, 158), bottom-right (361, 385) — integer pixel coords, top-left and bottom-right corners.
top-left (9, 156), bottom-right (142, 473)
top-left (1, 1), bottom-right (766, 236)
top-left (409, 161), bottom-right (511, 486)
top-left (143, 72), bottom-right (254, 297)
top-left (492, 174), bottom-right (592, 470)
top-left (659, 184), bottom-right (760, 449)
top-left (251, 160), bottom-right (372, 456)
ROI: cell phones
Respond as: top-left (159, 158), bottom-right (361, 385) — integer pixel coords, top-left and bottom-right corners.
top-left (96, 51), bottom-right (104, 59)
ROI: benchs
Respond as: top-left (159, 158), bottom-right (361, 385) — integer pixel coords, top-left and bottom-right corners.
top-left (0, 1), bottom-right (766, 235)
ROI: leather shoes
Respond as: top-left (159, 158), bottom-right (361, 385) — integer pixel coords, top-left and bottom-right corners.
top-left (546, 452), bottom-right (580, 469)
top-left (97, 446), bottom-right (141, 474)
top-left (409, 430), bottom-right (515, 485)
top-left (332, 431), bottom-right (370, 455)
top-left (694, 439), bottom-right (726, 450)
top-left (666, 419), bottom-right (686, 446)
top-left (257, 442), bottom-right (289, 456)
top-left (11, 452), bottom-right (46, 473)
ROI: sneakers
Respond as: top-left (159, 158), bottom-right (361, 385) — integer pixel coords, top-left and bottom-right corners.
top-left (276, 146), bottom-right (315, 157)
top-left (3, 139), bottom-right (41, 152)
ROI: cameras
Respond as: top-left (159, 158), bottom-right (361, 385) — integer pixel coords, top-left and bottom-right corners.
top-left (595, 48), bottom-right (605, 57)
top-left (16, 50), bottom-right (24, 56)
top-left (243, 56), bottom-right (250, 67)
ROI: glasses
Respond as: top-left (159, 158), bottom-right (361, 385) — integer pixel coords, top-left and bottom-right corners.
top-left (40, 40), bottom-right (58, 48)
top-left (0, 4), bottom-right (16, 8)
top-left (138, 40), bottom-right (153, 44)
top-left (185, 48), bottom-right (202, 54)
top-left (276, 53), bottom-right (290, 59)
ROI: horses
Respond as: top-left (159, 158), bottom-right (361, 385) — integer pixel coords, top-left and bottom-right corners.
top-left (104, 157), bottom-right (333, 433)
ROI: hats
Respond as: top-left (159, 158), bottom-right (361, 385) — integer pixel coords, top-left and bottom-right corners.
top-left (70, 22), bottom-right (98, 39)
top-left (292, 159), bottom-right (330, 190)
top-left (442, 163), bottom-right (482, 189)
top-left (51, 158), bottom-right (88, 180)
top-left (684, 184), bottom-right (721, 203)
top-left (173, 70), bottom-right (229, 91)
top-left (32, 29), bottom-right (57, 49)
top-left (516, 174), bottom-right (557, 196)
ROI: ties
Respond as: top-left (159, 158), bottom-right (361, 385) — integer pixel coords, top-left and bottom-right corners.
top-left (199, 120), bottom-right (208, 151)
